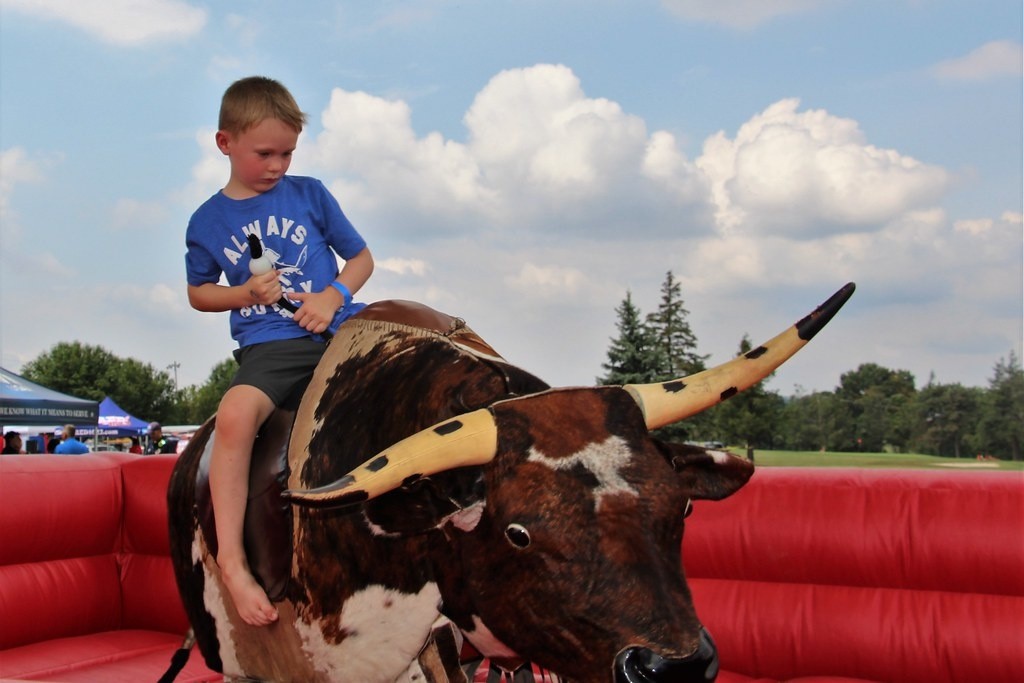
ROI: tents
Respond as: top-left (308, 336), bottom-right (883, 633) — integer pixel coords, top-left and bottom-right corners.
top-left (0, 367), bottom-right (100, 453)
top-left (54, 396), bottom-right (152, 450)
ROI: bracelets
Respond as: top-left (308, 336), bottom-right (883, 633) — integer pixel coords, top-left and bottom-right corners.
top-left (329, 281), bottom-right (354, 313)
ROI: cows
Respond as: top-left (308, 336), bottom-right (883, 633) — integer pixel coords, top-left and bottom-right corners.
top-left (157, 282), bottom-right (856, 683)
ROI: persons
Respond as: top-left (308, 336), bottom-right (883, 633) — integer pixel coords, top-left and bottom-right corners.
top-left (40, 431), bottom-right (143, 454)
top-left (54, 424), bottom-right (89, 455)
top-left (2, 432), bottom-right (22, 454)
top-left (183, 77), bottom-right (376, 627)
top-left (145, 422), bottom-right (176, 455)
